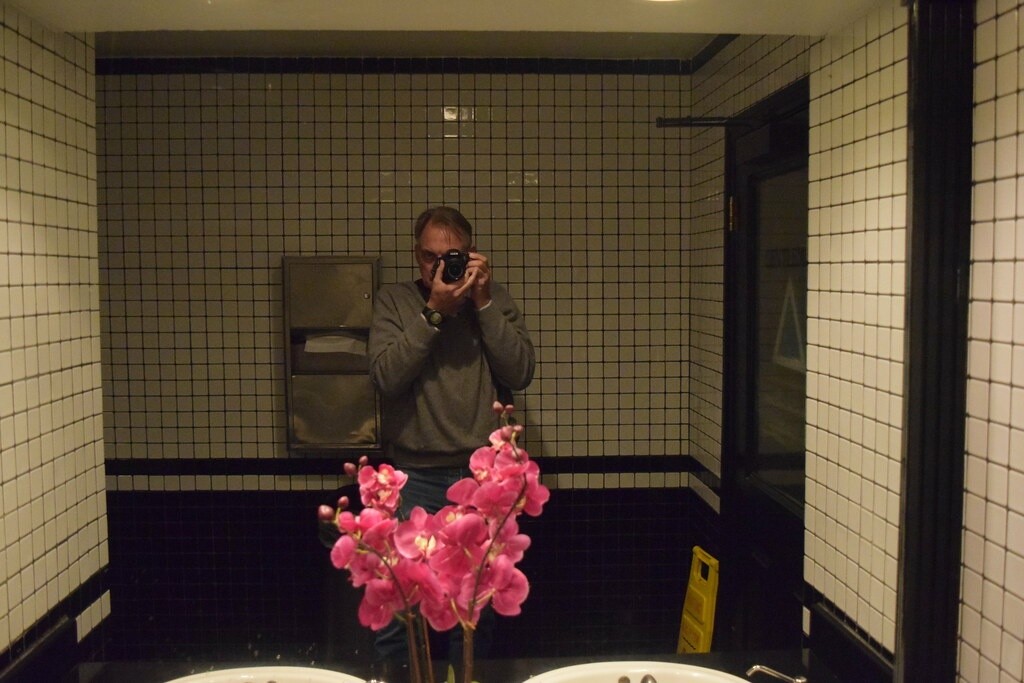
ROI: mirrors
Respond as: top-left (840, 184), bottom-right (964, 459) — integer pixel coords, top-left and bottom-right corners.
top-left (0, 0), bottom-right (974, 683)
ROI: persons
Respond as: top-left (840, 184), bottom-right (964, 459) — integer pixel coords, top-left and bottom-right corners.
top-left (367, 207), bottom-right (535, 683)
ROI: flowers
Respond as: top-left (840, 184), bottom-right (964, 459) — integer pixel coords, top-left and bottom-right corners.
top-left (315, 401), bottom-right (550, 683)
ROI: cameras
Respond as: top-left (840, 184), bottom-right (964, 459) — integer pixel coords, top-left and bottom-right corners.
top-left (431, 249), bottom-right (473, 283)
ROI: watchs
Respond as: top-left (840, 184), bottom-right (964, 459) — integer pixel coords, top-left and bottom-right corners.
top-left (422, 307), bottom-right (445, 327)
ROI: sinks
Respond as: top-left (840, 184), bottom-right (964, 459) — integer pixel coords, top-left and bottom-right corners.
top-left (162, 664), bottom-right (366, 683)
top-left (522, 660), bottom-right (752, 683)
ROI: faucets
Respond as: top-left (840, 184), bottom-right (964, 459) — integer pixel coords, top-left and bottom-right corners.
top-left (746, 664), bottom-right (808, 683)
top-left (618, 675), bottom-right (630, 683)
